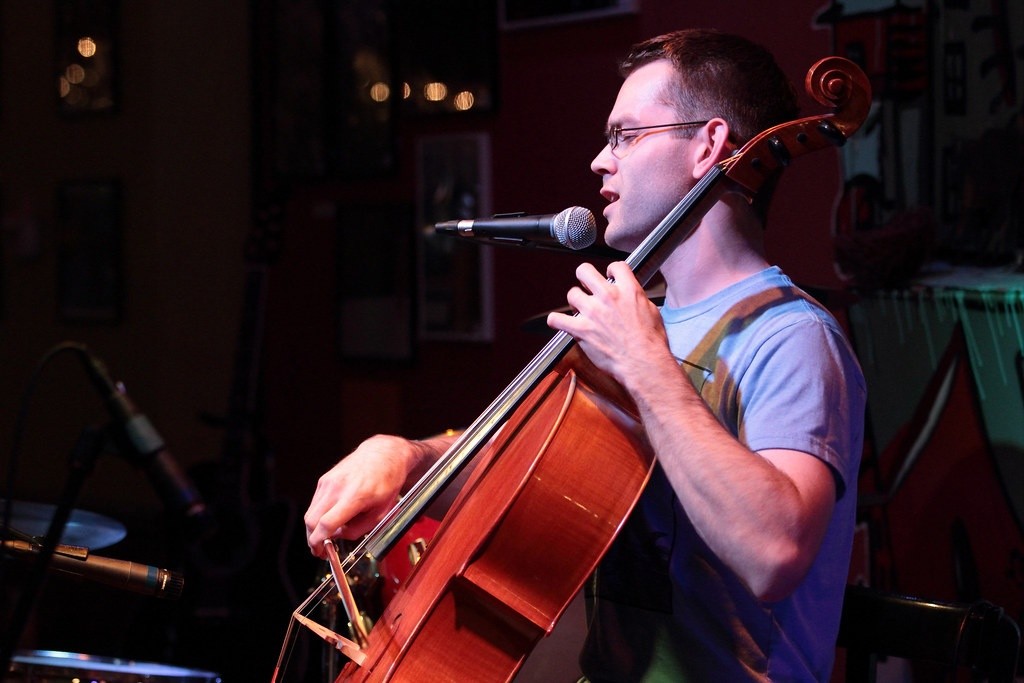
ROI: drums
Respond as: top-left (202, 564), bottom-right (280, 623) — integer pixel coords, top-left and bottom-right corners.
top-left (5, 645), bottom-right (223, 683)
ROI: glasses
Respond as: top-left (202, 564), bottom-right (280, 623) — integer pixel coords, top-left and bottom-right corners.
top-left (607, 120), bottom-right (737, 150)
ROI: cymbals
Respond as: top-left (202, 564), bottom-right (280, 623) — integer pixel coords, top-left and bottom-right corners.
top-left (0, 503), bottom-right (130, 552)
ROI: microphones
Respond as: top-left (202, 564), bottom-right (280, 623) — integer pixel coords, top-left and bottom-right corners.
top-left (76, 345), bottom-right (207, 520)
top-left (0, 538), bottom-right (188, 601)
top-left (434, 206), bottom-right (597, 251)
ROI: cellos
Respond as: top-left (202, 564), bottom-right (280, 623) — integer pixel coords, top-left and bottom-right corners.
top-left (267, 56), bottom-right (873, 683)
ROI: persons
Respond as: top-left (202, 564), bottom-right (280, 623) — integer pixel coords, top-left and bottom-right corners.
top-left (299, 24), bottom-right (871, 683)
